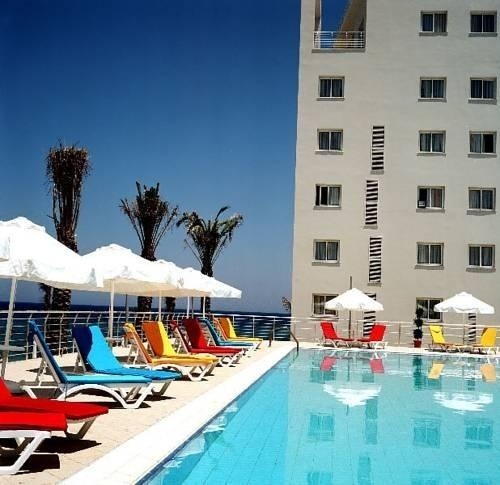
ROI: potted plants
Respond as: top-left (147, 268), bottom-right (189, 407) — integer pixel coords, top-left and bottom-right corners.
top-left (413, 308), bottom-right (425, 348)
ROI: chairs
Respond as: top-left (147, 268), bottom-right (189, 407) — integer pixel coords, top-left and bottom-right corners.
top-left (427, 324), bottom-right (497, 354)
top-left (428, 359), bottom-right (497, 382)
top-left (319, 353), bottom-right (387, 377)
top-left (319, 322), bottom-right (388, 349)
top-left (0, 315), bottom-right (264, 474)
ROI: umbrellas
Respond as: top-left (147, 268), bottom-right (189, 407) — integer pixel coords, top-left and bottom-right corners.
top-left (324, 356), bottom-right (382, 408)
top-left (324, 288), bottom-right (384, 339)
top-left (161, 399), bottom-right (239, 469)
top-left (434, 362), bottom-right (493, 414)
top-left (433, 290), bottom-right (495, 345)
